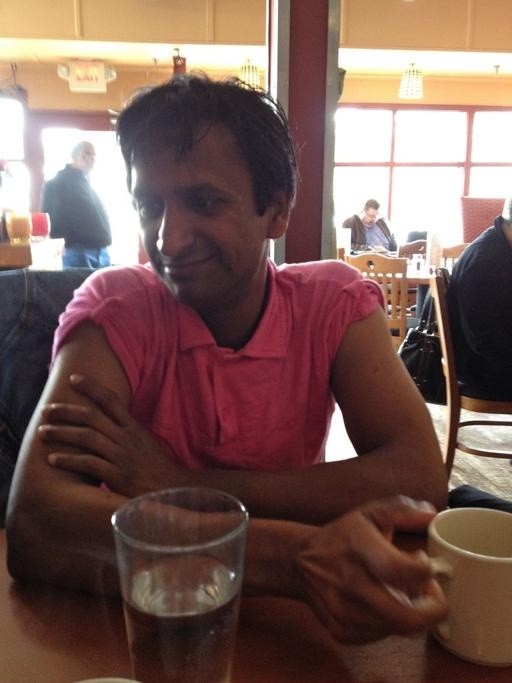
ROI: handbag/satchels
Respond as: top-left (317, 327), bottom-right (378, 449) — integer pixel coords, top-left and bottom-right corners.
top-left (390, 322), bottom-right (448, 405)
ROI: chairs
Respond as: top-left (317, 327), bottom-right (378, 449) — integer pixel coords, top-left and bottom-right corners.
top-left (338, 248), bottom-right (344, 260)
top-left (397, 239), bottom-right (426, 261)
top-left (346, 253), bottom-right (407, 350)
top-left (441, 244), bottom-right (471, 267)
top-left (429, 268), bottom-right (512, 486)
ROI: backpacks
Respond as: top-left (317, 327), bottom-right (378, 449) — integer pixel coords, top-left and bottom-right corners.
top-left (40, 177), bottom-right (69, 239)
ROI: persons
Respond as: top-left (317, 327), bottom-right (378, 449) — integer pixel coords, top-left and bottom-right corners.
top-left (40, 140), bottom-right (113, 270)
top-left (447, 195), bottom-right (512, 414)
top-left (6, 68), bottom-right (447, 643)
top-left (341, 199), bottom-right (397, 251)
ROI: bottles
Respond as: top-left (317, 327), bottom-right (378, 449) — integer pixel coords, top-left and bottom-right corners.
top-left (31, 212), bottom-right (52, 244)
top-left (10, 214), bottom-right (32, 244)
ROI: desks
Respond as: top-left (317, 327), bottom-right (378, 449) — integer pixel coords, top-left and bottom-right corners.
top-left (1, 266), bottom-right (101, 480)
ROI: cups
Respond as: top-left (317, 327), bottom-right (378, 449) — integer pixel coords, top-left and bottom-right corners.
top-left (424, 508), bottom-right (512, 668)
top-left (111, 487), bottom-right (249, 683)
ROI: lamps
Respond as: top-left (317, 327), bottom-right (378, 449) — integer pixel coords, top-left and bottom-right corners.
top-left (397, 58), bottom-right (423, 99)
top-left (238, 54), bottom-right (259, 91)
